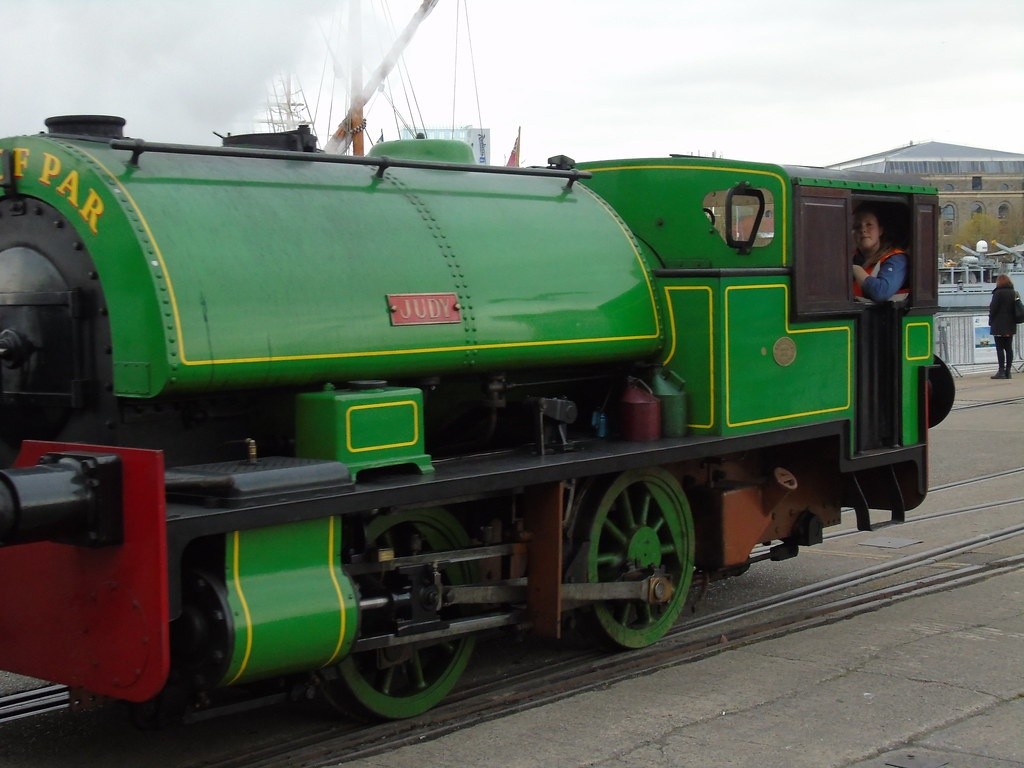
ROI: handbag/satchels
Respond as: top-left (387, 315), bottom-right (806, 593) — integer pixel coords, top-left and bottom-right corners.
top-left (1014, 291), bottom-right (1024, 324)
top-left (854, 266), bottom-right (909, 304)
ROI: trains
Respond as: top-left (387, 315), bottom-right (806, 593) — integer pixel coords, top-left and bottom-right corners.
top-left (0, 116), bottom-right (955, 723)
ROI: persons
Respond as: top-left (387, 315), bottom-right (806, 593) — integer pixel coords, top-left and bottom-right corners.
top-left (833, 210), bottom-right (909, 302)
top-left (989, 275), bottom-right (1020, 379)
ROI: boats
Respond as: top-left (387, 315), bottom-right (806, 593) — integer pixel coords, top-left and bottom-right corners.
top-left (937, 264), bottom-right (1020, 308)
top-left (979, 334), bottom-right (991, 346)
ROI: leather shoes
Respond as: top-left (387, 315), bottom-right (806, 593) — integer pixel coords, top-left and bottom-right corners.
top-left (990, 370), bottom-right (1011, 380)
